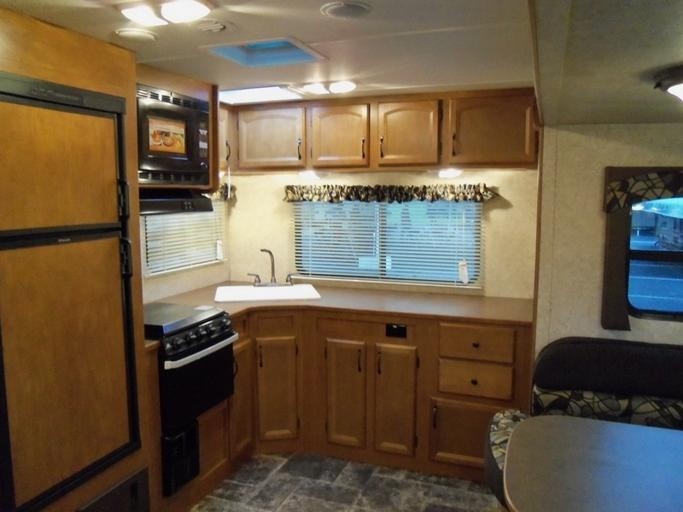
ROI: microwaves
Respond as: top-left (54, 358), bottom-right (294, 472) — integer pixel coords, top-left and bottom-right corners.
top-left (137, 83), bottom-right (209, 185)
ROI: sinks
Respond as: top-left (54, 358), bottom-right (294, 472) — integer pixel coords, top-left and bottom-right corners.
top-left (215, 283), bottom-right (321, 305)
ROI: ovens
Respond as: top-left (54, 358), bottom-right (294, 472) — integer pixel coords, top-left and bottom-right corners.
top-left (143, 301), bottom-right (238, 418)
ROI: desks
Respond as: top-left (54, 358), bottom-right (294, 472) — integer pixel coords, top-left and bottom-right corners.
top-left (497, 415), bottom-right (683, 510)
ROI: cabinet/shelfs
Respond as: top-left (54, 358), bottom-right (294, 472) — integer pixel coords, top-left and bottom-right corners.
top-left (217, 100), bottom-right (233, 172)
top-left (253, 306), bottom-right (309, 458)
top-left (445, 88), bottom-right (540, 171)
top-left (425, 313), bottom-right (540, 486)
top-left (310, 94), bottom-right (445, 173)
top-left (309, 306), bottom-right (425, 479)
top-left (228, 100), bottom-right (310, 170)
top-left (225, 308), bottom-right (257, 476)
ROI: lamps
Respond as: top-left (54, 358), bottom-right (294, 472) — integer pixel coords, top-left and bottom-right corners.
top-left (110, 1), bottom-right (219, 30)
top-left (650, 63), bottom-right (683, 105)
top-left (297, 81), bottom-right (353, 95)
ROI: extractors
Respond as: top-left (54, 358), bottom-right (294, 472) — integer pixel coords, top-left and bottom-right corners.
top-left (138, 188), bottom-right (213, 215)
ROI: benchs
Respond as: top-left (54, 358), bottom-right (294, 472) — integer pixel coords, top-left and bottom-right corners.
top-left (484, 334), bottom-right (682, 511)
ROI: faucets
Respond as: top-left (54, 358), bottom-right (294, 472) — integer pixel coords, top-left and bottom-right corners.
top-left (260, 248), bottom-right (276, 283)
